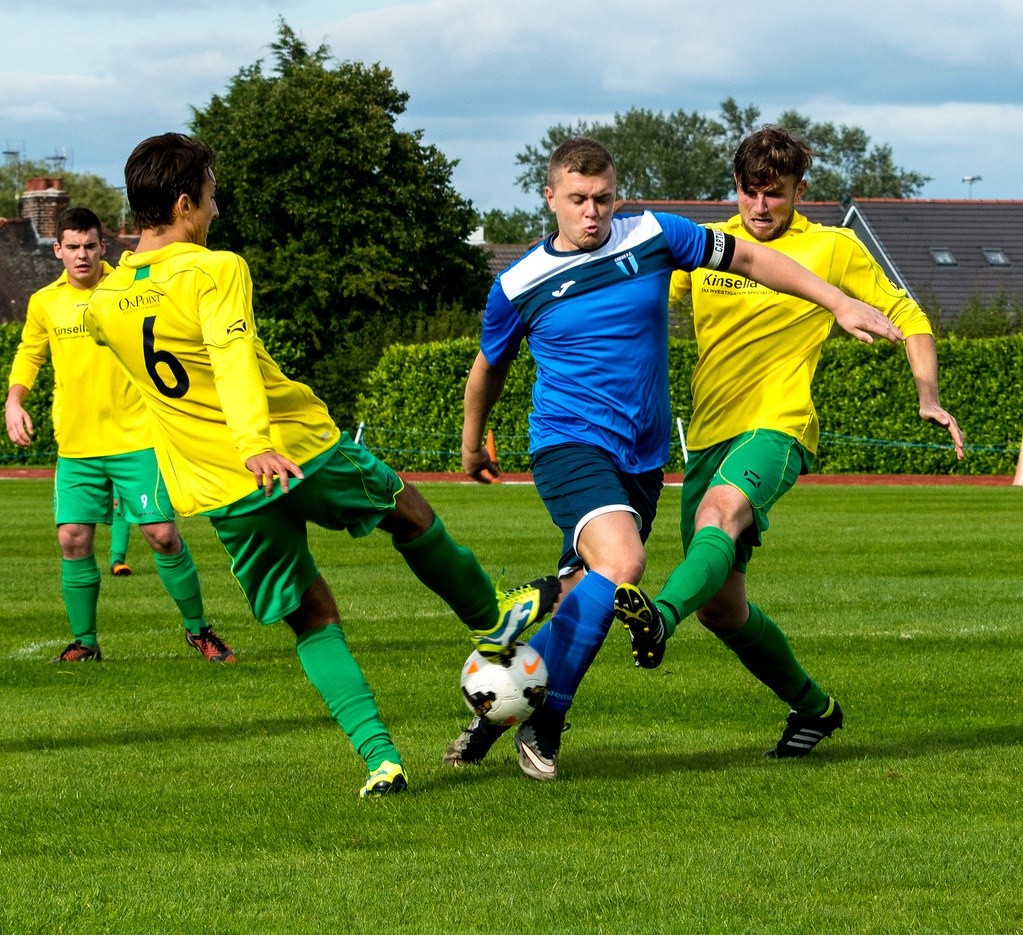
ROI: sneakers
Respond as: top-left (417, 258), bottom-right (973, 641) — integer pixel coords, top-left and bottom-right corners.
top-left (466, 573), bottom-right (563, 669)
top-left (765, 695), bottom-right (845, 759)
top-left (613, 584), bottom-right (667, 668)
top-left (514, 721), bottom-right (558, 782)
top-left (47, 639), bottom-right (103, 667)
top-left (442, 711), bottom-right (510, 771)
top-left (358, 759), bottom-right (409, 797)
top-left (185, 626), bottom-right (235, 665)
top-left (111, 559), bottom-right (131, 576)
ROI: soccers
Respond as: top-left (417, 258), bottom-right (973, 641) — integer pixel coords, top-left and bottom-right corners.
top-left (460, 640), bottom-right (549, 728)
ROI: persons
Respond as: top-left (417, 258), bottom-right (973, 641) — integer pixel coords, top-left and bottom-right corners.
top-left (443, 137), bottom-right (905, 780)
top-left (613, 119), bottom-right (965, 759)
top-left (110, 486), bottom-right (133, 576)
top-left (85, 133), bottom-right (562, 798)
top-left (5, 207), bottom-right (235, 663)
